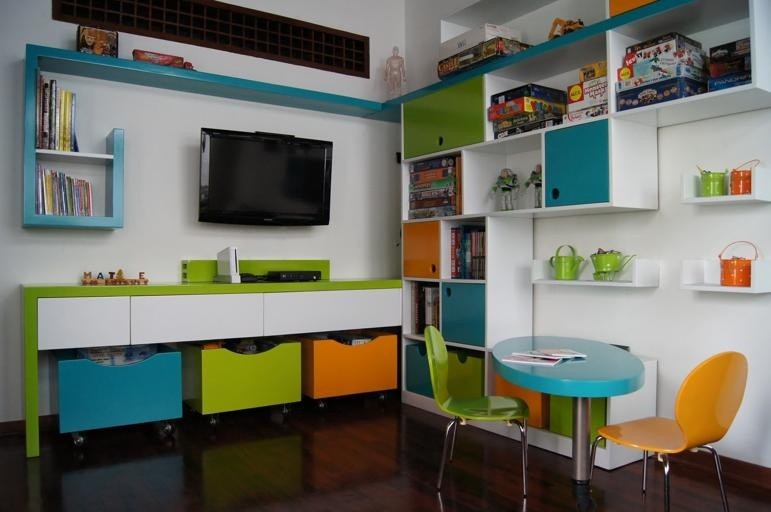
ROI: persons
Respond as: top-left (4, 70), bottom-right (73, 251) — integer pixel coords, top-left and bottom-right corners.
top-left (383, 46), bottom-right (407, 100)
top-left (489, 165), bottom-right (543, 210)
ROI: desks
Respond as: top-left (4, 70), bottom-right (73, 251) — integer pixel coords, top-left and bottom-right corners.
top-left (491, 337), bottom-right (649, 510)
top-left (20, 280), bottom-right (401, 459)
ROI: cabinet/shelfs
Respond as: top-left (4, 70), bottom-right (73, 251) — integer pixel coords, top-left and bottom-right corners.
top-left (398, 0), bottom-right (765, 470)
top-left (23, 41), bottom-right (124, 230)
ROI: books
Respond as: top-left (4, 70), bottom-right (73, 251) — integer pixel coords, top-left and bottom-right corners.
top-left (411, 282), bottom-right (440, 335)
top-left (449, 226), bottom-right (486, 280)
top-left (36, 163), bottom-right (94, 216)
top-left (36, 70), bottom-right (80, 153)
top-left (501, 346), bottom-right (587, 366)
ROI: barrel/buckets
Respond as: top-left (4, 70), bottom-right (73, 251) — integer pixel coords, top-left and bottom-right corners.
top-left (590, 250), bottom-right (625, 271)
top-left (729, 159), bottom-right (761, 195)
top-left (700, 167), bottom-right (729, 196)
top-left (718, 240), bottom-right (758, 287)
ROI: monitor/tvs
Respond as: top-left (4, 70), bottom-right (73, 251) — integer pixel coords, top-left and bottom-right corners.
top-left (198, 127), bottom-right (334, 226)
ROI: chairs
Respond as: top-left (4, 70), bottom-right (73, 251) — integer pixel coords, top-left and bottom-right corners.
top-left (422, 325), bottom-right (530, 504)
top-left (588, 350), bottom-right (746, 512)
